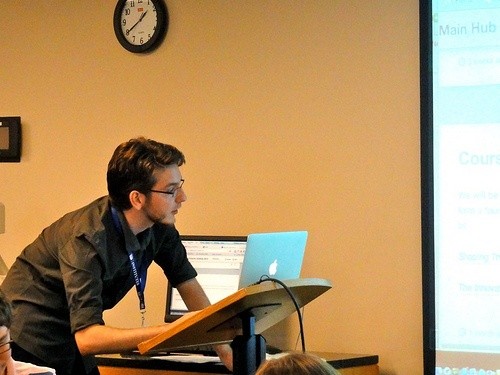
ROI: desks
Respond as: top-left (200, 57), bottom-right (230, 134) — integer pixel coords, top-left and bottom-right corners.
top-left (95, 351), bottom-right (380, 375)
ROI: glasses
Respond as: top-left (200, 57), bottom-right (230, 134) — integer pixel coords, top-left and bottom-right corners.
top-left (0, 332), bottom-right (14, 355)
top-left (144, 178), bottom-right (184, 197)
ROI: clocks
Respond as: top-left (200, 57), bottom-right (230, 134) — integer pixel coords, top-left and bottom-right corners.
top-left (113, 0), bottom-right (168, 54)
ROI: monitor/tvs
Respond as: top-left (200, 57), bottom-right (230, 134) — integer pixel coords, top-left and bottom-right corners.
top-left (164, 235), bottom-right (247, 323)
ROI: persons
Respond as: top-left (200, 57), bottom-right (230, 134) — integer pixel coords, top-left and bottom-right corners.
top-left (0, 137), bottom-right (233, 375)
top-left (255, 353), bottom-right (341, 375)
top-left (0, 293), bottom-right (57, 375)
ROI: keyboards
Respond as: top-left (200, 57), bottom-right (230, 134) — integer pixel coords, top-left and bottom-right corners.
top-left (119, 342), bottom-right (219, 357)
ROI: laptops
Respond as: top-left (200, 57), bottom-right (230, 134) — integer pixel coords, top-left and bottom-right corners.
top-left (238, 231), bottom-right (308, 291)
